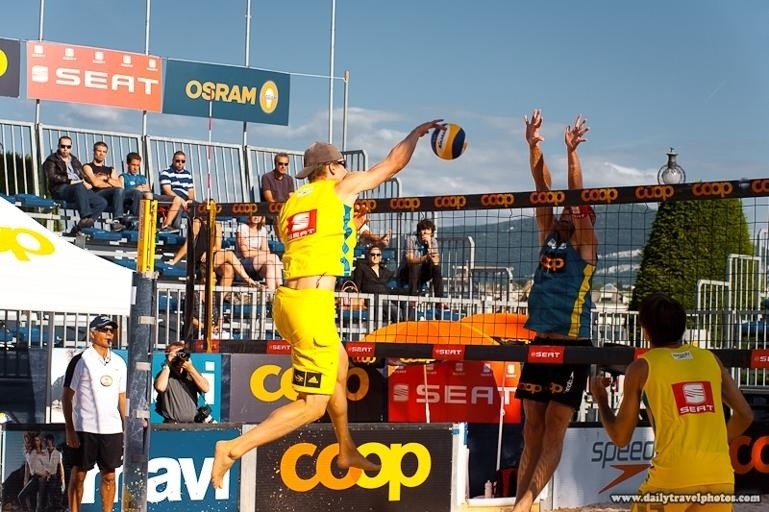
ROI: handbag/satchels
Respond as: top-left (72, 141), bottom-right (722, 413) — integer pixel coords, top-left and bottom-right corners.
top-left (335, 281), bottom-right (366, 312)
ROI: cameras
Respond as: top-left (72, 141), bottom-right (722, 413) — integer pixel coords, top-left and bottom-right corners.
top-left (172, 349), bottom-right (191, 368)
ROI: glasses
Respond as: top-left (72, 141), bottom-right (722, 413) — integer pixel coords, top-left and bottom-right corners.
top-left (100, 329), bottom-right (115, 335)
top-left (175, 160), bottom-right (185, 162)
top-left (60, 145), bottom-right (72, 149)
top-left (339, 161), bottom-right (346, 167)
top-left (370, 254), bottom-right (381, 256)
top-left (278, 162), bottom-right (288, 166)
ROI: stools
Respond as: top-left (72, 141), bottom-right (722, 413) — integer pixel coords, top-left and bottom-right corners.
top-left (500, 468), bottom-right (520, 497)
top-left (1, 186), bottom-right (462, 348)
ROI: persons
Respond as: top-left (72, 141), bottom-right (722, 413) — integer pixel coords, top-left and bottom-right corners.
top-left (153, 340), bottom-right (210, 423)
top-left (44, 135), bottom-right (450, 349)
top-left (590, 292), bottom-right (754, 512)
top-left (210, 117), bottom-right (447, 491)
top-left (61, 315), bottom-right (128, 511)
top-left (17, 432), bottom-right (67, 512)
top-left (515, 108), bottom-right (599, 512)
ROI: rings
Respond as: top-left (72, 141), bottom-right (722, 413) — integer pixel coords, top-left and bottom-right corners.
top-left (66, 443), bottom-right (69, 445)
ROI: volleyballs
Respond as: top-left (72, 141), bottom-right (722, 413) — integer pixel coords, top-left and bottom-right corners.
top-left (431, 125), bottom-right (467, 160)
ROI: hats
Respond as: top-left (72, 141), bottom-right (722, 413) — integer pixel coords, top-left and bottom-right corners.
top-left (90, 315), bottom-right (117, 330)
top-left (294, 142), bottom-right (341, 179)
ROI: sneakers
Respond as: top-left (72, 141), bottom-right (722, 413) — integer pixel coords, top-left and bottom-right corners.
top-left (80, 217), bottom-right (94, 226)
top-left (111, 222), bottom-right (125, 230)
top-left (159, 226), bottom-right (180, 234)
